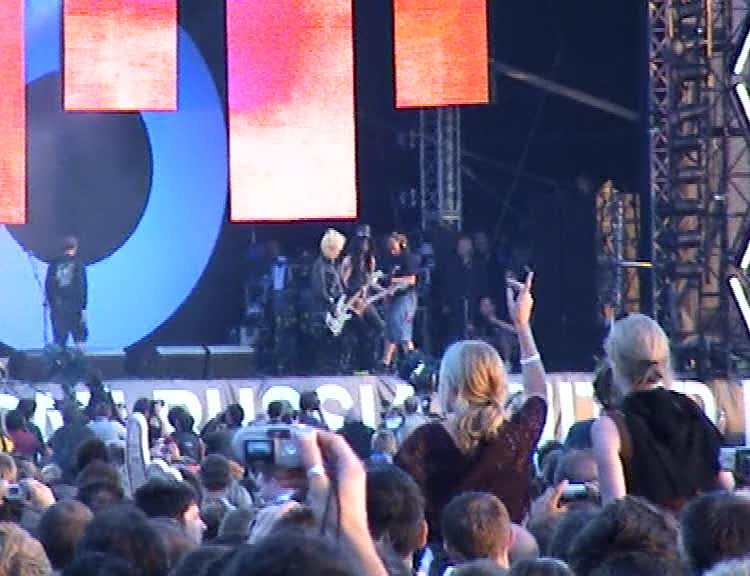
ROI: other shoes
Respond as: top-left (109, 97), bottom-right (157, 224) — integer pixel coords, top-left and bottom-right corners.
top-left (371, 361), bottom-right (394, 375)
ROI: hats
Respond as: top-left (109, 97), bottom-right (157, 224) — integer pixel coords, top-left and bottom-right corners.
top-left (352, 223), bottom-right (374, 239)
top-left (64, 236), bottom-right (79, 250)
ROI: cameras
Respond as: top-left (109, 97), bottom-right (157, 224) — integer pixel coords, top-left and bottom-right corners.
top-left (719, 448), bottom-right (750, 471)
top-left (561, 486), bottom-right (590, 505)
top-left (233, 427), bottom-right (319, 468)
top-left (5, 484), bottom-right (29, 501)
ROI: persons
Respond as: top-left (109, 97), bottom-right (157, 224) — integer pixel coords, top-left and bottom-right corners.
top-left (0, 366), bottom-right (750, 576)
top-left (590, 313), bottom-right (734, 508)
top-left (392, 271), bottom-right (549, 543)
top-left (45, 235), bottom-right (90, 357)
top-left (311, 223), bottom-right (531, 373)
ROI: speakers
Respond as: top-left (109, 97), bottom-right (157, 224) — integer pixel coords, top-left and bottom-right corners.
top-left (136, 344), bottom-right (206, 380)
top-left (8, 349), bottom-right (55, 379)
top-left (207, 346), bottom-right (255, 378)
top-left (68, 346), bottom-right (127, 376)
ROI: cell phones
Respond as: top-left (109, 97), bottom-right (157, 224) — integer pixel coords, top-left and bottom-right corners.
top-left (381, 416), bottom-right (404, 430)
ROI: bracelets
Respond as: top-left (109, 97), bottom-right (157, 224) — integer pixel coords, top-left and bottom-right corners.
top-left (520, 353), bottom-right (541, 365)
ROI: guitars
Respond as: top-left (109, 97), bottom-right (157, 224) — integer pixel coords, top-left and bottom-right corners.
top-left (325, 270), bottom-right (384, 336)
top-left (351, 275), bottom-right (410, 316)
